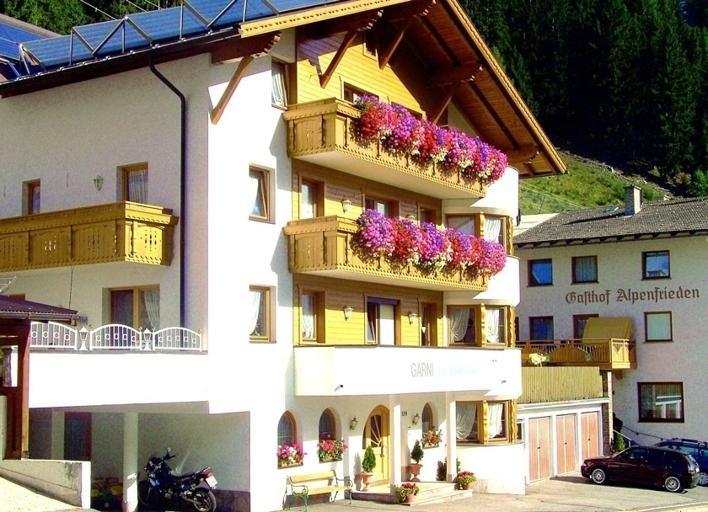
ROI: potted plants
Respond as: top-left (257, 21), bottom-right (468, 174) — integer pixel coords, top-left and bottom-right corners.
top-left (407, 439), bottom-right (426, 483)
top-left (354, 445), bottom-right (377, 492)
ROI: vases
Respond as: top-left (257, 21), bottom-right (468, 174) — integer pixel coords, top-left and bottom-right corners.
top-left (463, 483), bottom-right (473, 490)
top-left (405, 493), bottom-right (415, 503)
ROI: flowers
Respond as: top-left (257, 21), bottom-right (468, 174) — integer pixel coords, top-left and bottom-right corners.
top-left (394, 482), bottom-right (419, 501)
top-left (278, 441), bottom-right (303, 466)
top-left (353, 92), bottom-right (509, 186)
top-left (456, 469), bottom-right (475, 489)
top-left (318, 432), bottom-right (348, 460)
top-left (355, 206), bottom-right (506, 281)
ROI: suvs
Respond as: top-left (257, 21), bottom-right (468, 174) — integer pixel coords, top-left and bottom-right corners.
top-left (628, 437), bottom-right (707, 485)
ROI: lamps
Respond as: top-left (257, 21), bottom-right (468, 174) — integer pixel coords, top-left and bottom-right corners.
top-left (412, 412), bottom-right (420, 425)
top-left (406, 310), bottom-right (418, 325)
top-left (349, 415), bottom-right (358, 432)
top-left (91, 173), bottom-right (105, 191)
top-left (343, 304), bottom-right (354, 320)
top-left (340, 192), bottom-right (352, 212)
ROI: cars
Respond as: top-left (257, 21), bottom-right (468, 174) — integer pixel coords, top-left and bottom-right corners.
top-left (580, 445), bottom-right (700, 495)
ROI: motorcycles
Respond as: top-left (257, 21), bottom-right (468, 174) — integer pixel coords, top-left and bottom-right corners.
top-left (139, 447), bottom-right (222, 511)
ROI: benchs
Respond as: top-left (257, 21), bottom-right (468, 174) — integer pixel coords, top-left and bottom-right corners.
top-left (285, 469), bottom-right (357, 511)
